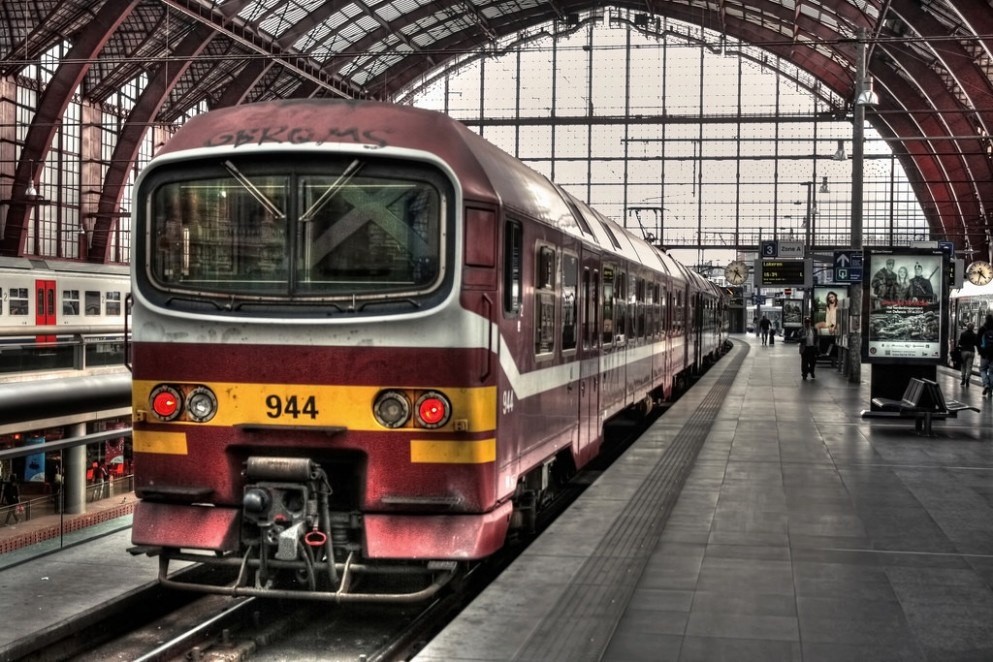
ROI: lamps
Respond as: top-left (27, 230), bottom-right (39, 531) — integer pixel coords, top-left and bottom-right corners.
top-left (25, 160), bottom-right (36, 195)
top-left (857, 70), bottom-right (879, 105)
top-left (833, 140), bottom-right (847, 161)
top-left (819, 177), bottom-right (831, 193)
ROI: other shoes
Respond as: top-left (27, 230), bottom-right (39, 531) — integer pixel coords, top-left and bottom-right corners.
top-left (4, 523), bottom-right (11, 526)
top-left (810, 371), bottom-right (815, 378)
top-left (988, 390), bottom-right (992, 398)
top-left (803, 375), bottom-right (806, 380)
top-left (982, 384), bottom-right (989, 395)
top-left (16, 519), bottom-right (21, 524)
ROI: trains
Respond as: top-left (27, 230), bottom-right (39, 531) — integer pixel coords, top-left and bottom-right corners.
top-left (0, 257), bottom-right (132, 341)
top-left (122, 98), bottom-right (735, 608)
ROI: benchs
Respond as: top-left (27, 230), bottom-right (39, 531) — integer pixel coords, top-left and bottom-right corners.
top-left (817, 343), bottom-right (834, 368)
top-left (872, 377), bottom-right (980, 437)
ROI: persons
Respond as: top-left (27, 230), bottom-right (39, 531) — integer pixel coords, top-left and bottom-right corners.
top-left (49, 464), bottom-right (60, 503)
top-left (753, 316), bottom-right (771, 345)
top-left (816, 292), bottom-right (838, 333)
top-left (2, 474), bottom-right (21, 525)
top-left (798, 317), bottom-right (819, 381)
top-left (957, 322), bottom-right (977, 390)
top-left (359, 225), bottom-right (392, 268)
top-left (91, 462), bottom-right (107, 501)
top-left (873, 255), bottom-right (934, 302)
top-left (976, 314), bottom-right (993, 399)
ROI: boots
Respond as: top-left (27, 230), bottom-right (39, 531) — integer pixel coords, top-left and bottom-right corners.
top-left (966, 378), bottom-right (969, 387)
top-left (960, 375), bottom-right (965, 385)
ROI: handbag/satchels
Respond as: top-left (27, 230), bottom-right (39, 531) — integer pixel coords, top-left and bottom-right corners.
top-left (15, 505), bottom-right (24, 515)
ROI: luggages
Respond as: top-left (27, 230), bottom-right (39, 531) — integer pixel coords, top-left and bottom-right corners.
top-left (769, 327), bottom-right (775, 345)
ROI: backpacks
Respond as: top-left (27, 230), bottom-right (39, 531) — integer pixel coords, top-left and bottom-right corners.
top-left (950, 347), bottom-right (964, 364)
top-left (980, 325), bottom-right (993, 350)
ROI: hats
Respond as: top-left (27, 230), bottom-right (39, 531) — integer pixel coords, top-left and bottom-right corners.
top-left (886, 259), bottom-right (894, 264)
top-left (804, 316), bottom-right (811, 321)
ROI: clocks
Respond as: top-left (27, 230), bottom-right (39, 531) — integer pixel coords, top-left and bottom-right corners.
top-left (724, 261), bottom-right (748, 285)
top-left (966, 261), bottom-right (993, 285)
